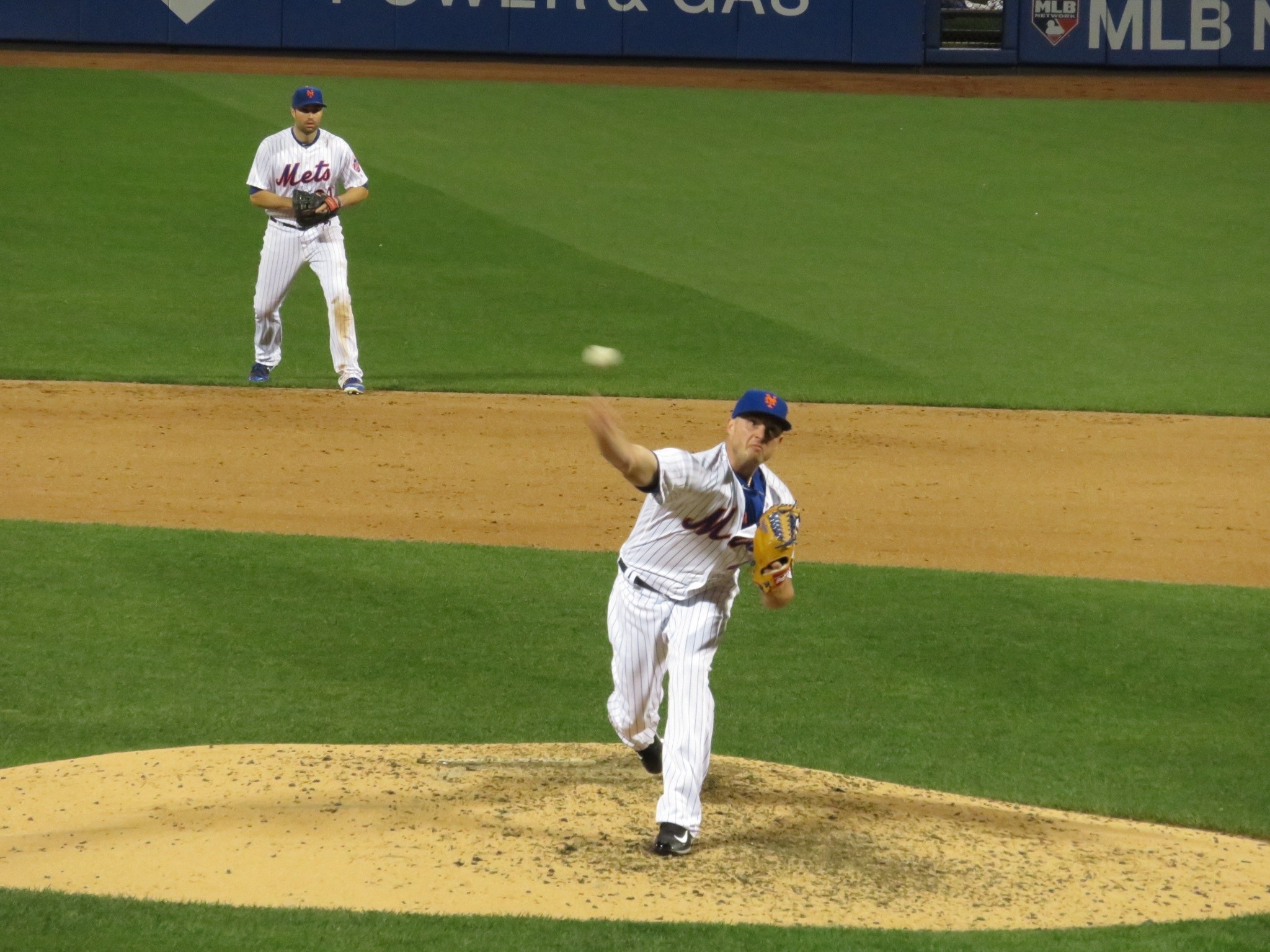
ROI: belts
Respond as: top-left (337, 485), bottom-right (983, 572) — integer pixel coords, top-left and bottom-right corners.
top-left (270, 216), bottom-right (306, 231)
top-left (618, 558), bottom-right (659, 593)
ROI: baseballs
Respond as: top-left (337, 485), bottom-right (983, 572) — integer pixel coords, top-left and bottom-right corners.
top-left (582, 343), bottom-right (618, 368)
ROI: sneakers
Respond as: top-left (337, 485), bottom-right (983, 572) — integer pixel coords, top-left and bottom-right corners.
top-left (248, 363), bottom-right (274, 381)
top-left (655, 822), bottom-right (694, 856)
top-left (635, 733), bottom-right (662, 774)
top-left (344, 378), bottom-right (365, 395)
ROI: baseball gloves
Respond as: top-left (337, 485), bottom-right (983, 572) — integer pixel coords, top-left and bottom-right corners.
top-left (750, 500), bottom-right (802, 594)
top-left (292, 188), bottom-right (339, 231)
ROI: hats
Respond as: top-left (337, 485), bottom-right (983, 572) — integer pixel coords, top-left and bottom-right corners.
top-left (292, 85), bottom-right (327, 109)
top-left (731, 389), bottom-right (792, 431)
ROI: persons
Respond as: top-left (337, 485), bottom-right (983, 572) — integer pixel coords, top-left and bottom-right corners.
top-left (249, 88), bottom-right (368, 395)
top-left (580, 392), bottom-right (800, 853)
